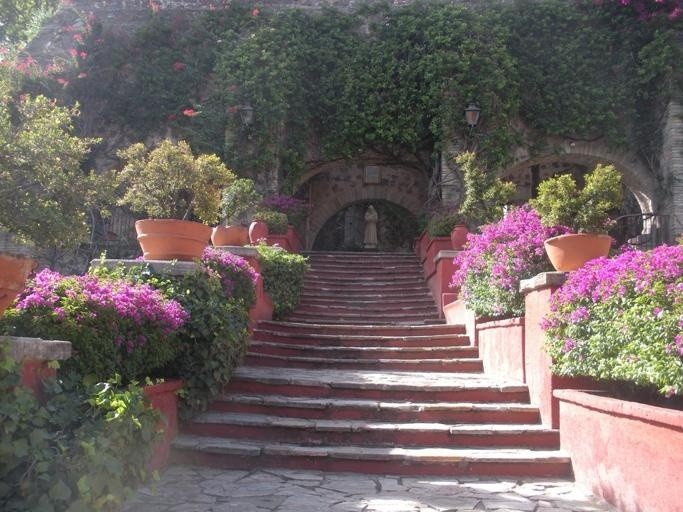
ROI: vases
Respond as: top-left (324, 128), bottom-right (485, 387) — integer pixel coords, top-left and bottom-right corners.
top-left (449, 223), bottom-right (469, 251)
top-left (249, 218), bottom-right (269, 246)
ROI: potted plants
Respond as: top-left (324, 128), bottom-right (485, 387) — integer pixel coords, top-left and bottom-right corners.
top-left (114, 139), bottom-right (227, 260)
top-left (209, 177), bottom-right (263, 246)
top-left (530, 163), bottom-right (624, 271)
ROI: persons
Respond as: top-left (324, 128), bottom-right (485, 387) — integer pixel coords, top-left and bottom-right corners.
top-left (362, 204), bottom-right (382, 248)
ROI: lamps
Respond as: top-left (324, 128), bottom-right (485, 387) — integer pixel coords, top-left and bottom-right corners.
top-left (462, 102), bottom-right (481, 135)
top-left (236, 103), bottom-right (258, 141)
